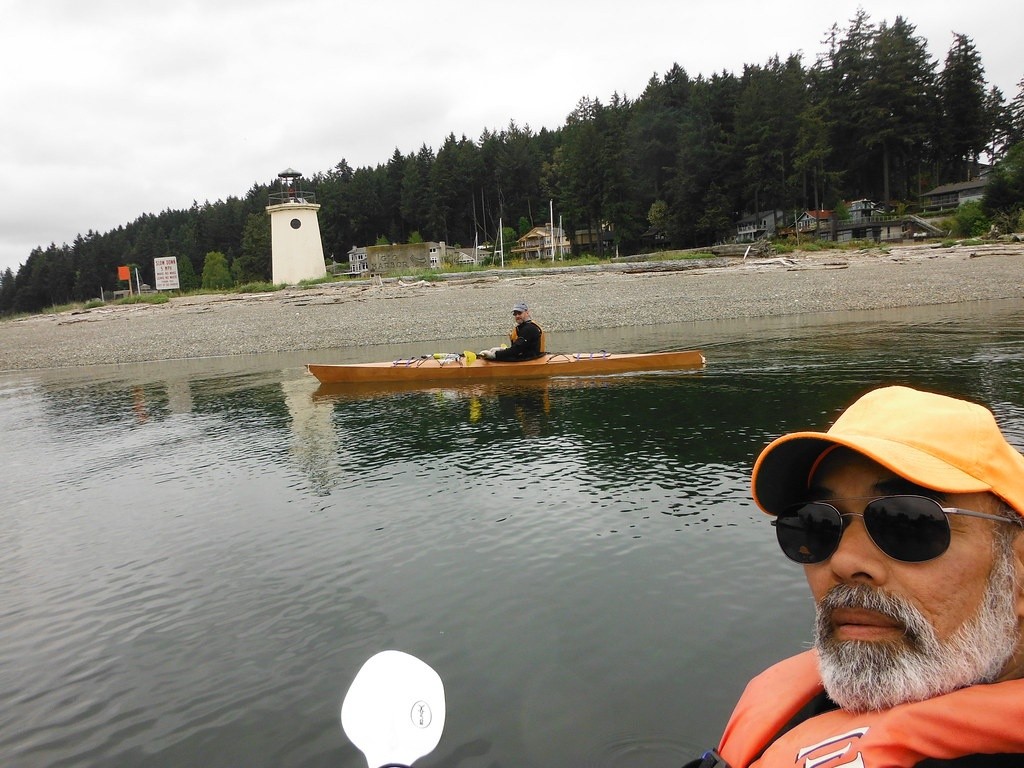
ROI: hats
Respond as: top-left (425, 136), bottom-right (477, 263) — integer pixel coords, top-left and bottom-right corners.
top-left (511, 304), bottom-right (528, 313)
top-left (751, 386), bottom-right (1024, 518)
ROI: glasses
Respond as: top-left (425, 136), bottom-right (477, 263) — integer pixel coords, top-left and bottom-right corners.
top-left (771, 495), bottom-right (1024, 566)
top-left (513, 312), bottom-right (522, 316)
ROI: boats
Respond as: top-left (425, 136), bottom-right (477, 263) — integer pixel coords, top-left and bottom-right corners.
top-left (308, 350), bottom-right (707, 380)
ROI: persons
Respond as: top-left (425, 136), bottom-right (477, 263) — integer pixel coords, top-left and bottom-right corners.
top-left (680, 385), bottom-right (1023, 768)
top-left (484, 304), bottom-right (546, 362)
